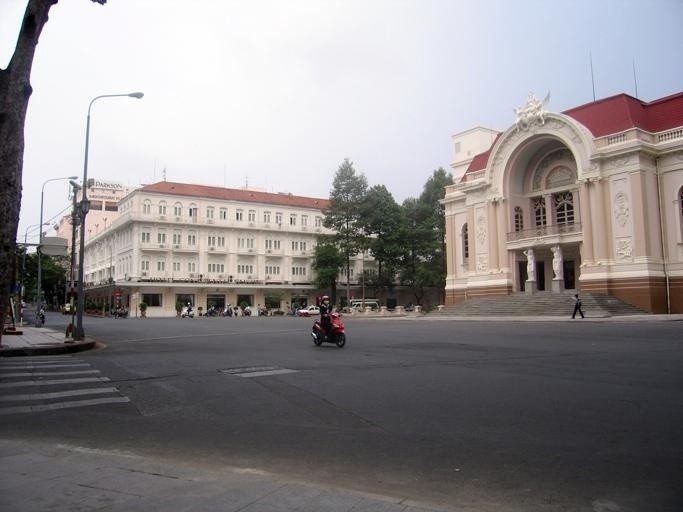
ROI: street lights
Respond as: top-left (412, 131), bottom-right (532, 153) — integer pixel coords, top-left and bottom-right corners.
top-left (35, 174), bottom-right (77, 313)
top-left (93, 239), bottom-right (113, 318)
top-left (21, 222), bottom-right (51, 290)
top-left (68, 89), bottom-right (146, 343)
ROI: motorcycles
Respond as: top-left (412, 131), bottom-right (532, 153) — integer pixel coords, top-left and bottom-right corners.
top-left (310, 311), bottom-right (346, 348)
top-left (180, 304), bottom-right (268, 318)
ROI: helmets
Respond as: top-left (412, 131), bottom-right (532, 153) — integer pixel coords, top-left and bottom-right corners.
top-left (322, 295), bottom-right (329, 300)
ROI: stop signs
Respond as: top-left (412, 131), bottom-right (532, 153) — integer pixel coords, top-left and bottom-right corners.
top-left (113, 290), bottom-right (121, 298)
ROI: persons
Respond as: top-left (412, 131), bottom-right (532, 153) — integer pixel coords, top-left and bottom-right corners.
top-left (40, 305), bottom-right (45, 324)
top-left (319, 295), bottom-right (333, 338)
top-left (572, 294), bottom-right (584, 319)
top-left (552, 252), bottom-right (562, 280)
top-left (187, 303), bottom-right (267, 316)
top-left (523, 249), bottom-right (536, 281)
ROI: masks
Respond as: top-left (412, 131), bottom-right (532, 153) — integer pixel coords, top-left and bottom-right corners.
top-left (324, 301), bottom-right (329, 304)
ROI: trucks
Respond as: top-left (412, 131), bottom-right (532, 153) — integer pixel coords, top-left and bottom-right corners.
top-left (351, 301), bottom-right (379, 312)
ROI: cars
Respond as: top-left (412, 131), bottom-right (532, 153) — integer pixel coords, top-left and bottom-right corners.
top-left (296, 305), bottom-right (320, 315)
top-left (21, 301), bottom-right (26, 308)
top-left (61, 303), bottom-right (76, 315)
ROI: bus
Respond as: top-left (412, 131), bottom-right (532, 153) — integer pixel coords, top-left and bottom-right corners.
top-left (342, 298), bottom-right (381, 309)
